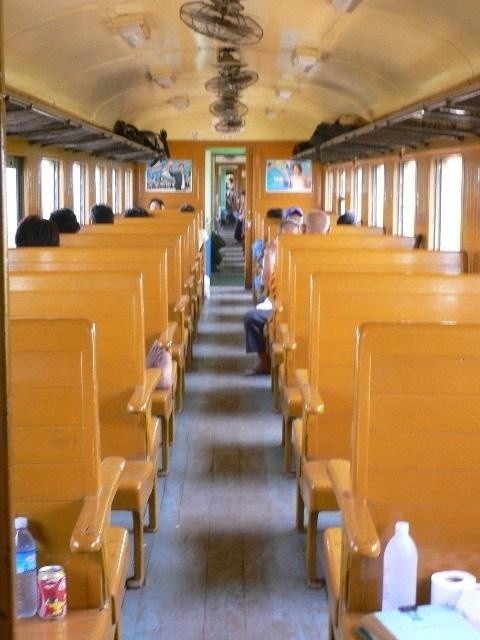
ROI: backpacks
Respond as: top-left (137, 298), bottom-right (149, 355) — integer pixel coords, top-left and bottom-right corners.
top-left (113, 119), bottom-right (171, 159)
top-left (308, 119), bottom-right (356, 159)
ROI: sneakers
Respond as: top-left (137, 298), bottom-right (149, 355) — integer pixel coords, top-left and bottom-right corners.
top-left (244, 367), bottom-right (271, 376)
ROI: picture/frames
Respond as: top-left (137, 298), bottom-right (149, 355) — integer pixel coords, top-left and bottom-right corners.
top-left (265, 159), bottom-right (312, 194)
top-left (145, 158), bottom-right (192, 193)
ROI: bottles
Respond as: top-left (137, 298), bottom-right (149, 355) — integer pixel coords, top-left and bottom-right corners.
top-left (382, 522), bottom-right (418, 610)
top-left (15, 517), bottom-right (38, 622)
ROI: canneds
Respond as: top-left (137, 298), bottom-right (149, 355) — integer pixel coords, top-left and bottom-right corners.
top-left (37, 565), bottom-right (68, 619)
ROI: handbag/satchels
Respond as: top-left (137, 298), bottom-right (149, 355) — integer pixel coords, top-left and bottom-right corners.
top-left (145, 340), bottom-right (171, 389)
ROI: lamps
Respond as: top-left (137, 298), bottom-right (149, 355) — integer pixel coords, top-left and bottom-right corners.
top-left (267, 1), bottom-right (359, 120)
top-left (116, 22), bottom-right (189, 109)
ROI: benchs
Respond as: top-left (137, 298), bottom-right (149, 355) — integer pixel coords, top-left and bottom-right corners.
top-left (264, 211), bottom-right (478, 639)
top-left (8, 210), bottom-right (203, 640)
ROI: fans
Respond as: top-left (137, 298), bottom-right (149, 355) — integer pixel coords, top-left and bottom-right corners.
top-left (179, 0), bottom-right (263, 131)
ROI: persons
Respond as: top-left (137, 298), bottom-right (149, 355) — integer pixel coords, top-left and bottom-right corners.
top-left (169, 162), bottom-right (189, 191)
top-left (290, 163), bottom-right (307, 190)
top-left (49, 208), bottom-right (80, 234)
top-left (15, 215), bottom-right (60, 247)
top-left (90, 205), bottom-right (114, 223)
top-left (211, 178), bottom-right (357, 377)
top-left (179, 204), bottom-right (194, 212)
top-left (149, 199), bottom-right (165, 211)
top-left (123, 207), bottom-right (148, 218)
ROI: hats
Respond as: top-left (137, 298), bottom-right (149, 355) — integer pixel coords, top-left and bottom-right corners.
top-left (284, 206), bottom-right (304, 218)
